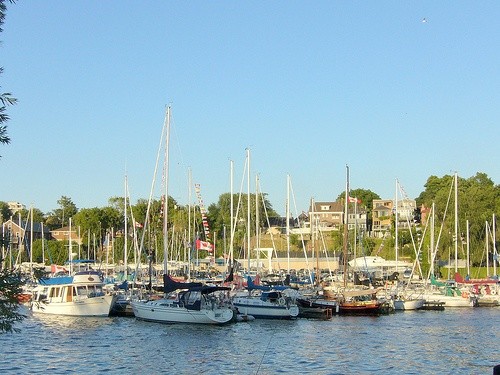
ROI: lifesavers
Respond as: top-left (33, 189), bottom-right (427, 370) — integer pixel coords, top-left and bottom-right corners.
top-left (462, 292), bottom-right (468, 298)
top-left (279, 298), bottom-right (286, 305)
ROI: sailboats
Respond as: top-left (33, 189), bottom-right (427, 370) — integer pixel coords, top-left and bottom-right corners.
top-left (1, 104), bottom-right (500, 324)
